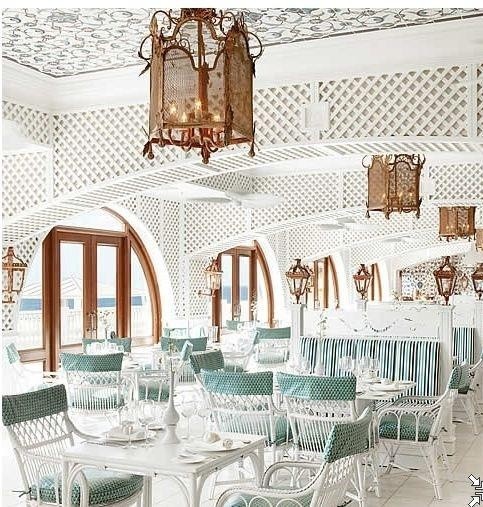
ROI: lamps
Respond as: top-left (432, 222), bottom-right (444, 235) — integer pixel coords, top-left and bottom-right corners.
top-left (353, 263), bottom-right (372, 295)
top-left (287, 260), bottom-right (310, 304)
top-left (361, 152), bottom-right (427, 220)
top-left (198, 260), bottom-right (224, 299)
top-left (137, 8), bottom-right (264, 164)
top-left (470, 264), bottom-right (482, 299)
top-left (439, 207), bottom-right (477, 240)
top-left (1, 246), bottom-right (28, 308)
top-left (436, 257), bottom-right (459, 306)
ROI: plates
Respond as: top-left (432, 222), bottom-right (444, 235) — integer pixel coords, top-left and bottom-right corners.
top-left (193, 437), bottom-right (241, 451)
top-left (369, 383), bottom-right (402, 391)
top-left (100, 430), bottom-right (156, 442)
top-left (176, 455), bottom-right (205, 463)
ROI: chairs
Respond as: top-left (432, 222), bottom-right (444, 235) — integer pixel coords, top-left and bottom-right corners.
top-left (202, 370), bottom-right (287, 446)
top-left (376, 367), bottom-right (452, 501)
top-left (159, 336), bottom-right (210, 384)
top-left (58, 350), bottom-right (136, 415)
top-left (216, 406), bottom-right (374, 506)
top-left (9, 344), bottom-right (58, 384)
top-left (457, 359), bottom-right (480, 435)
top-left (226, 320), bottom-right (246, 329)
top-left (187, 345), bottom-right (227, 378)
top-left (278, 372), bottom-right (381, 501)
top-left (255, 326), bottom-right (294, 369)
top-left (4, 384), bottom-right (150, 506)
top-left (83, 338), bottom-right (133, 359)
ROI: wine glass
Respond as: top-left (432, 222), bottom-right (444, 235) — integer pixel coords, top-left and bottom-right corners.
top-left (86, 341), bottom-right (125, 354)
top-left (138, 400), bottom-right (156, 448)
top-left (337, 356), bottom-right (380, 378)
top-left (197, 399), bottom-right (211, 437)
top-left (121, 408), bottom-right (139, 449)
top-left (180, 394), bottom-right (197, 439)
